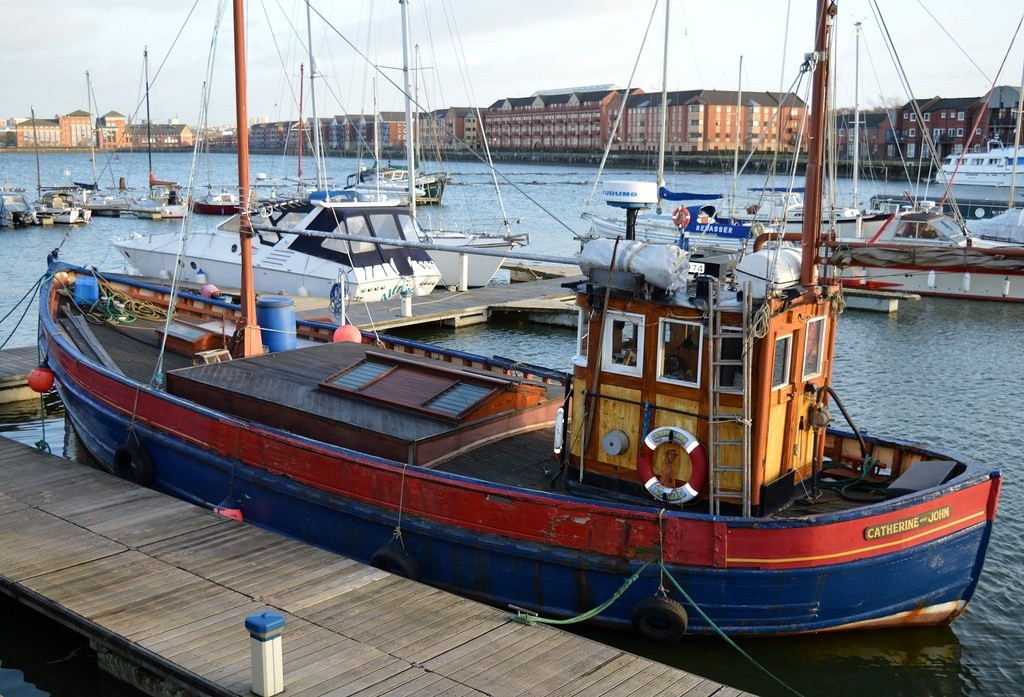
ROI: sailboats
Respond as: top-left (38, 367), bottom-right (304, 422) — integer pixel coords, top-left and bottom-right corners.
top-left (0, 0), bottom-right (531, 304)
top-left (32, 0), bottom-right (1004, 637)
top-left (568, 0), bottom-right (1024, 311)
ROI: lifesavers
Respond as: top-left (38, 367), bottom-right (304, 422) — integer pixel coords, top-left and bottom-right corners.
top-left (672, 207), bottom-right (690, 228)
top-left (637, 425), bottom-right (708, 506)
top-left (370, 544), bottom-right (424, 582)
top-left (631, 596), bottom-right (688, 642)
top-left (113, 443), bottom-right (159, 491)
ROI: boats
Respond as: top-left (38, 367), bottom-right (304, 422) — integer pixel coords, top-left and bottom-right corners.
top-left (932, 133), bottom-right (1024, 187)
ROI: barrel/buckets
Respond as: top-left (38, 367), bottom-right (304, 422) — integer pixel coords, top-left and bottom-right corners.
top-left (255, 296), bottom-right (296, 352)
